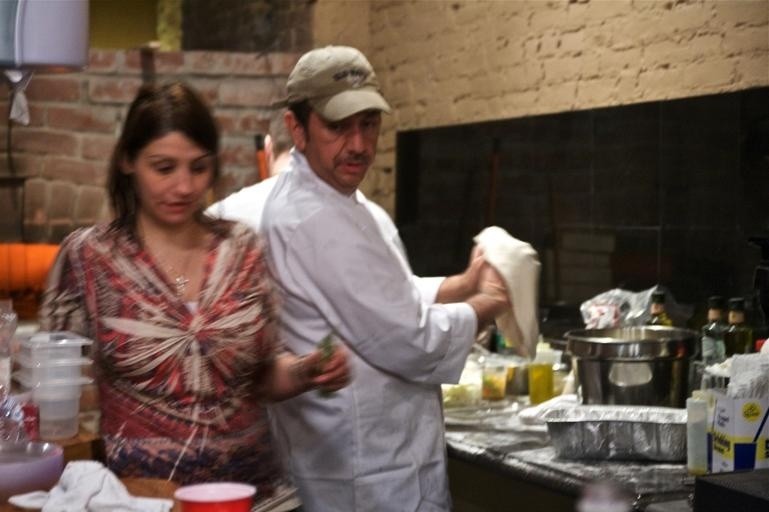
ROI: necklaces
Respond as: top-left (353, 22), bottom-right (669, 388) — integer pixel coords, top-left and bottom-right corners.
top-left (134, 213), bottom-right (198, 300)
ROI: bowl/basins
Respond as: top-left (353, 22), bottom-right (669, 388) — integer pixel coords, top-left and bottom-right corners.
top-left (1, 439), bottom-right (66, 500)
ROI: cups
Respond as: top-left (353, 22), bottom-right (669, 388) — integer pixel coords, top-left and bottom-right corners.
top-left (172, 481), bottom-right (258, 512)
top-left (460, 341), bottom-right (571, 408)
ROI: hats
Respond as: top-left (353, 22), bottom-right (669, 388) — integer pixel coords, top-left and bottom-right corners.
top-left (286, 45), bottom-right (392, 122)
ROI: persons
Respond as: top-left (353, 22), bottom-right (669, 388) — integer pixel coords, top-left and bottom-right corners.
top-left (36, 78), bottom-right (355, 512)
top-left (200, 97), bottom-right (416, 317)
top-left (256, 43), bottom-right (517, 510)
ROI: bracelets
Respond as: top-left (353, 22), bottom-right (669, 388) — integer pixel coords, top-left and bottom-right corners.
top-left (287, 353), bottom-right (323, 396)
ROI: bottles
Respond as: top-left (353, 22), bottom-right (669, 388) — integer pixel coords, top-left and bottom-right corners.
top-left (724, 295), bottom-right (754, 357)
top-left (686, 390), bottom-right (711, 477)
top-left (646, 291), bottom-right (675, 328)
top-left (700, 294), bottom-right (729, 392)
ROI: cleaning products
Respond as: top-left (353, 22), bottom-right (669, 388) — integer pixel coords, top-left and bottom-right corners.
top-left (726, 296), bottom-right (752, 352)
top-left (700, 295), bottom-right (727, 365)
